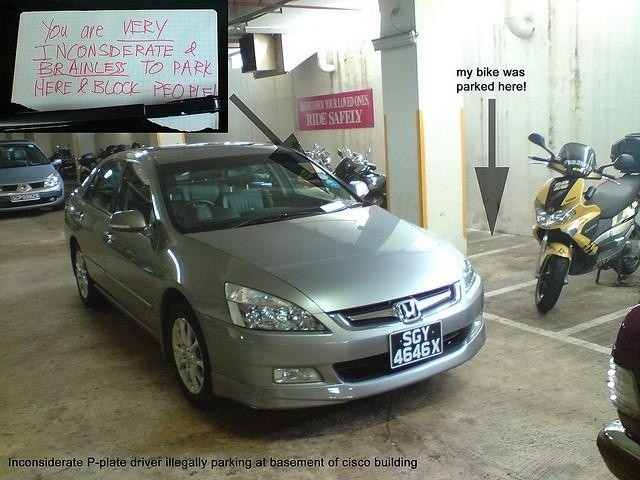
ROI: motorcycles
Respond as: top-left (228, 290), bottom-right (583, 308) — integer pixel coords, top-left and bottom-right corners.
top-left (40, 141), bottom-right (391, 214)
top-left (524, 127), bottom-right (640, 314)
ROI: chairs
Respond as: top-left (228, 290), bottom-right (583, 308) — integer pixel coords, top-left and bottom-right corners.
top-left (168, 167), bottom-right (269, 228)
top-left (1, 148), bottom-right (30, 165)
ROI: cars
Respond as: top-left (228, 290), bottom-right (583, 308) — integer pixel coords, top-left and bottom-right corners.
top-left (62, 140), bottom-right (487, 426)
top-left (0, 139), bottom-right (66, 221)
top-left (594, 297), bottom-right (639, 479)
